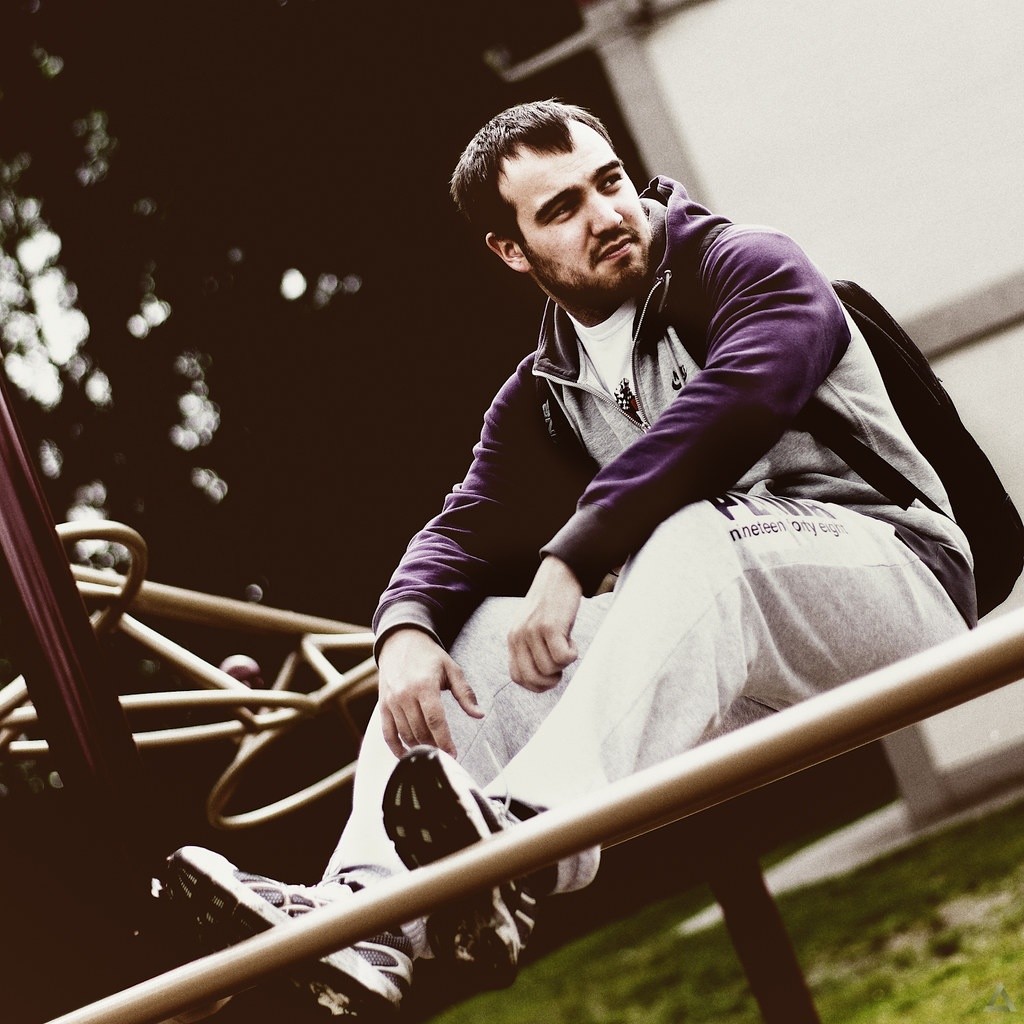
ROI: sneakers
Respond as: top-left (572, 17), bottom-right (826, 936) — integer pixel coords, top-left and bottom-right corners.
top-left (161, 846), bottom-right (414, 1024)
top-left (382, 745), bottom-right (537, 992)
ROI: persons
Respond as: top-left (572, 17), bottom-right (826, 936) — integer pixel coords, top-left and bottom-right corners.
top-left (164, 95), bottom-right (979, 1022)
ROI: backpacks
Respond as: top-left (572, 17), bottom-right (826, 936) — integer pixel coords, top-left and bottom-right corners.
top-left (537, 224), bottom-right (1024, 622)
